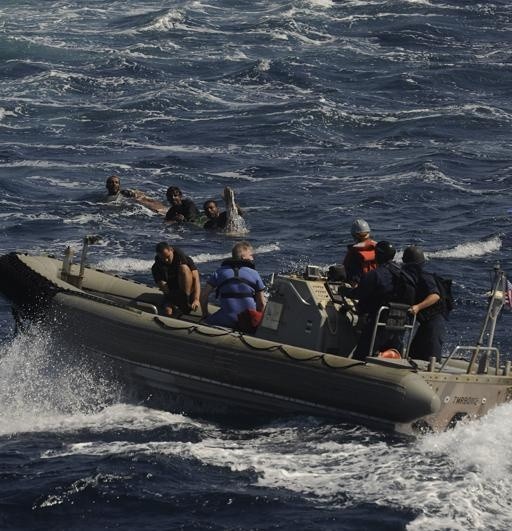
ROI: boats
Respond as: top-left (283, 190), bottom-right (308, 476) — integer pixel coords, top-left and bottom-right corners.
top-left (1, 231), bottom-right (512, 443)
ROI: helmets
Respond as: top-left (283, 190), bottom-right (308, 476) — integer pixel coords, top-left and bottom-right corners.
top-left (375, 241), bottom-right (396, 260)
top-left (403, 246), bottom-right (425, 266)
top-left (351, 219), bottom-right (371, 235)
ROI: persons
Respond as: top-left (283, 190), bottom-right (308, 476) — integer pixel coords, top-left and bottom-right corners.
top-left (150, 240), bottom-right (202, 318)
top-left (198, 241), bottom-right (266, 330)
top-left (342, 216), bottom-right (380, 285)
top-left (339, 238), bottom-right (416, 362)
top-left (402, 246), bottom-right (455, 364)
top-left (200, 184), bottom-right (246, 235)
top-left (104, 174), bottom-right (166, 215)
top-left (163, 184), bottom-right (200, 229)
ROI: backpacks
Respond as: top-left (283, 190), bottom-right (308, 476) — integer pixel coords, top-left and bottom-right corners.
top-left (391, 264), bottom-right (418, 305)
top-left (434, 271), bottom-right (457, 321)
